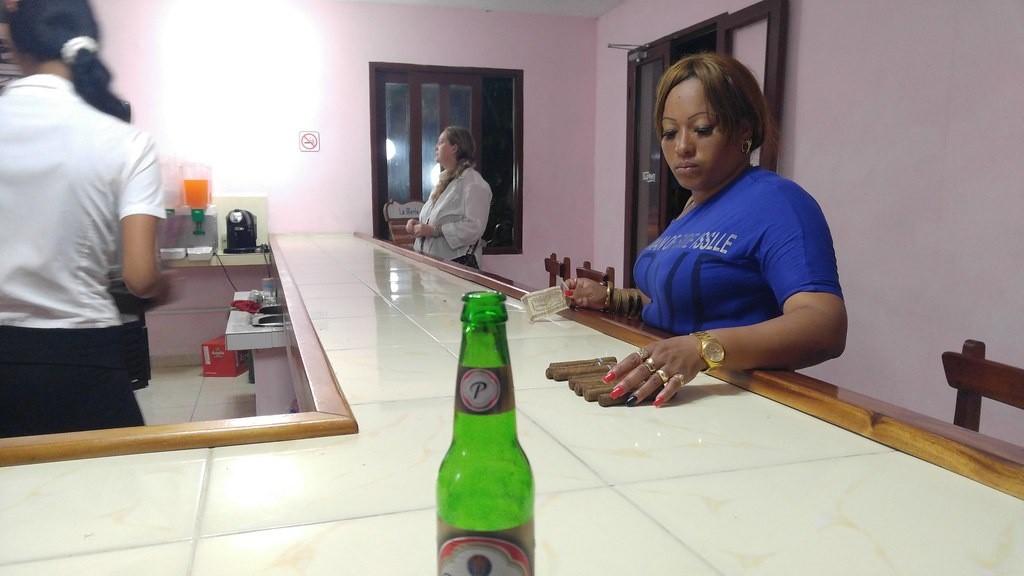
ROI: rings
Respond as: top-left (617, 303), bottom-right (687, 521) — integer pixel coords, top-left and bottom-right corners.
top-left (635, 346), bottom-right (650, 363)
top-left (656, 368), bottom-right (669, 382)
top-left (643, 357), bottom-right (658, 374)
top-left (673, 373), bottom-right (685, 386)
top-left (573, 278), bottom-right (578, 287)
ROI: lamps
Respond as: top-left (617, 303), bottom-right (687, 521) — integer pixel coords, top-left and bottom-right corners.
top-left (607, 41), bottom-right (649, 64)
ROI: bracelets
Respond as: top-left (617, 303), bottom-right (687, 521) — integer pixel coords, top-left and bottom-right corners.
top-left (603, 281), bottom-right (642, 319)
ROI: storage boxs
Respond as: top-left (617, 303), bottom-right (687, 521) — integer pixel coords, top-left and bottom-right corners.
top-left (201, 335), bottom-right (250, 377)
top-left (159, 247), bottom-right (185, 261)
top-left (186, 246), bottom-right (214, 261)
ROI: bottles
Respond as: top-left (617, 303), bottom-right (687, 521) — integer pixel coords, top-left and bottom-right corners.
top-left (435, 290), bottom-right (534, 576)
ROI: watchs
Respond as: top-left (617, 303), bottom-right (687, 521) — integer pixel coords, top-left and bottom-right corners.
top-left (689, 330), bottom-right (726, 374)
top-left (433, 224), bottom-right (439, 238)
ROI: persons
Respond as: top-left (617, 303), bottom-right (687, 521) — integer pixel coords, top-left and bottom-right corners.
top-left (405, 126), bottom-right (493, 271)
top-left (0, 0), bottom-right (183, 440)
top-left (564, 51), bottom-right (849, 409)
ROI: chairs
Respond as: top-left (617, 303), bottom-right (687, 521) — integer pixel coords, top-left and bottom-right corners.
top-left (576, 261), bottom-right (615, 288)
top-left (942, 340), bottom-right (1024, 432)
top-left (544, 253), bottom-right (570, 288)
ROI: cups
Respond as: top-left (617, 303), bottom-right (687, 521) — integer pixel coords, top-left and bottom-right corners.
top-left (262, 277), bottom-right (275, 305)
